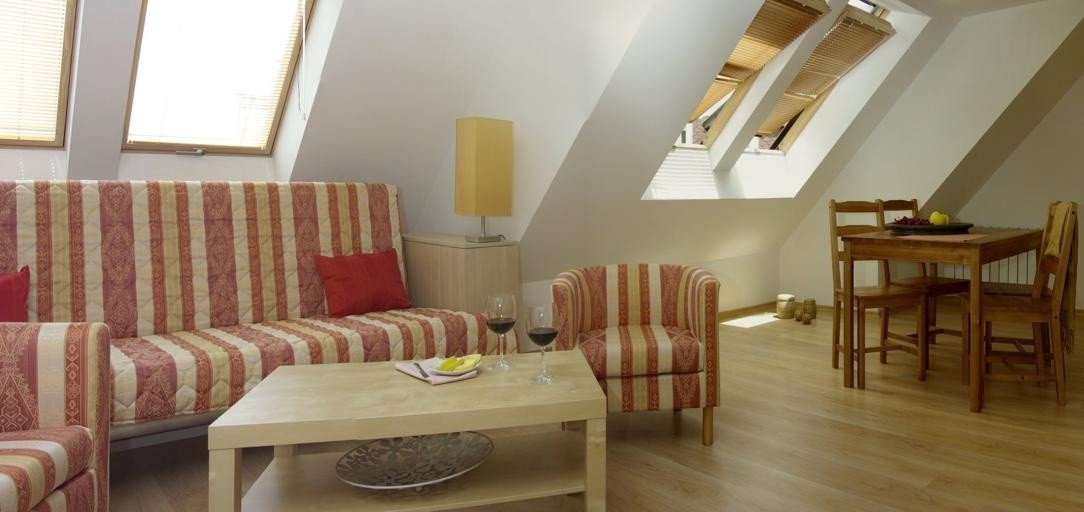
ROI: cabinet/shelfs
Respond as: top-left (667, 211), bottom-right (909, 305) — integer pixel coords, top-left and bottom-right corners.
top-left (404, 227), bottom-right (522, 349)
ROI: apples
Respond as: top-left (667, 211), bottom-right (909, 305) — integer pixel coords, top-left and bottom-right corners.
top-left (436, 353), bottom-right (482, 371)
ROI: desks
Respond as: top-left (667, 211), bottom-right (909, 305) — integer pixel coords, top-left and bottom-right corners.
top-left (840, 224), bottom-right (1052, 413)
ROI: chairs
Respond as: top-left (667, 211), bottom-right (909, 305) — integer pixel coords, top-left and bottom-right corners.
top-left (875, 197), bottom-right (975, 370)
top-left (960, 200), bottom-right (1076, 413)
top-left (830, 197), bottom-right (929, 391)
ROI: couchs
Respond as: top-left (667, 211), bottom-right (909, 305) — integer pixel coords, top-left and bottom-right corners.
top-left (0, 319), bottom-right (111, 512)
top-left (0, 180), bottom-right (518, 458)
top-left (549, 261), bottom-right (722, 445)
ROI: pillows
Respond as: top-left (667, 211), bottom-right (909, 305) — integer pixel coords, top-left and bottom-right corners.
top-left (315, 247), bottom-right (414, 318)
top-left (0, 266), bottom-right (31, 323)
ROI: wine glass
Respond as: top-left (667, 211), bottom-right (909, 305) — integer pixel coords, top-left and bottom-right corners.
top-left (524, 300), bottom-right (560, 384)
top-left (480, 290), bottom-right (518, 370)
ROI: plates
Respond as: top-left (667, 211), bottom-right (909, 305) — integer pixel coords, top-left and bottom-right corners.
top-left (434, 352), bottom-right (482, 376)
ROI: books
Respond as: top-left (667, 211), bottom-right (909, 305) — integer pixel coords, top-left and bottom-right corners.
top-left (897, 231), bottom-right (988, 243)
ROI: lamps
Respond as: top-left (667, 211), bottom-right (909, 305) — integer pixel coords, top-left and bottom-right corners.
top-left (453, 116), bottom-right (516, 245)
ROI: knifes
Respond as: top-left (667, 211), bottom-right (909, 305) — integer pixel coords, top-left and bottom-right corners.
top-left (414, 361), bottom-right (429, 379)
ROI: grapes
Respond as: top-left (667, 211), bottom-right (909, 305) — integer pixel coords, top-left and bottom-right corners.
top-left (891, 216), bottom-right (930, 225)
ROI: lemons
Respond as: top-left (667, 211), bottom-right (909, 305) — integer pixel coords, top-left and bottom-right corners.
top-left (930, 211), bottom-right (949, 225)
